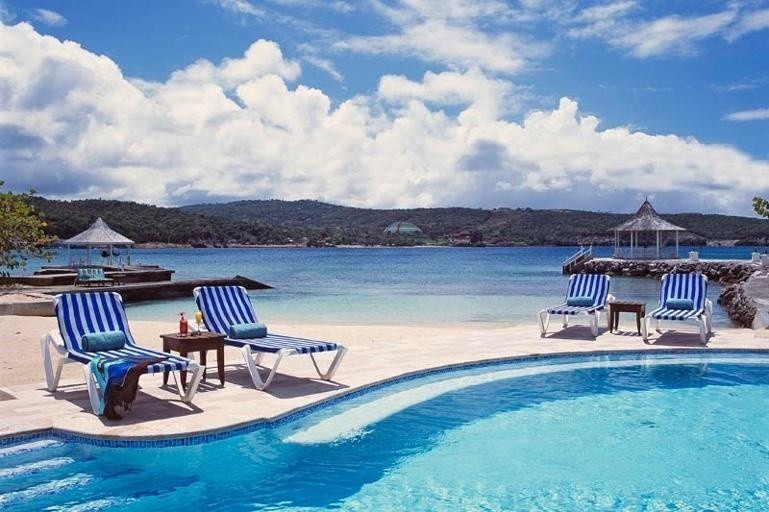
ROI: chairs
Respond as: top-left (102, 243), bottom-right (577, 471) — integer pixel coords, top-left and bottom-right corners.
top-left (192, 286), bottom-right (349, 390)
top-left (40, 291), bottom-right (207, 419)
top-left (534, 273), bottom-right (714, 344)
top-left (72, 267), bottom-right (115, 286)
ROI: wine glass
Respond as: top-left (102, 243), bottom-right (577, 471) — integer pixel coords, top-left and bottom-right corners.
top-left (195, 310), bottom-right (202, 334)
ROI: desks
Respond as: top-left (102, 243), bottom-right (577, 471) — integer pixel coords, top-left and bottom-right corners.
top-left (160, 331), bottom-right (226, 389)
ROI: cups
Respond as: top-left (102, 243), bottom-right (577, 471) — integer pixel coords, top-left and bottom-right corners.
top-left (180, 320), bottom-right (187, 336)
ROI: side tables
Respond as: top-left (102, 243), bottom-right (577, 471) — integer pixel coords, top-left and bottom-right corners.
top-left (110, 274), bottom-right (126, 285)
